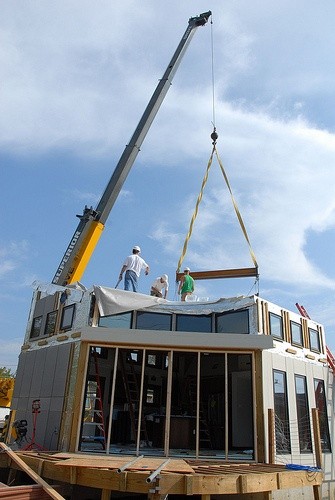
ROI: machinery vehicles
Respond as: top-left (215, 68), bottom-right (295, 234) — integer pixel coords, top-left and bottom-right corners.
top-left (0, 10), bottom-right (217, 445)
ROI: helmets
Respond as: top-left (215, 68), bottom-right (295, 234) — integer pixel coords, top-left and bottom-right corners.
top-left (184, 267), bottom-right (190, 271)
top-left (132, 246), bottom-right (140, 252)
top-left (161, 274), bottom-right (168, 282)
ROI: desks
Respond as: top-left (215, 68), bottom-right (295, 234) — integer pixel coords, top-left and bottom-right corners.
top-left (152, 415), bottom-right (201, 449)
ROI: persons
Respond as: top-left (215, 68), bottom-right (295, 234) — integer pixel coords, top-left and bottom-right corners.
top-left (178, 267), bottom-right (195, 301)
top-left (151, 274), bottom-right (168, 299)
top-left (119, 246), bottom-right (149, 292)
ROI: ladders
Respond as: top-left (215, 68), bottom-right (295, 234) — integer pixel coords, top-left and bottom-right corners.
top-left (78, 346), bottom-right (108, 452)
top-left (120, 352), bottom-right (149, 450)
top-left (187, 360), bottom-right (215, 451)
top-left (295, 303), bottom-right (335, 373)
